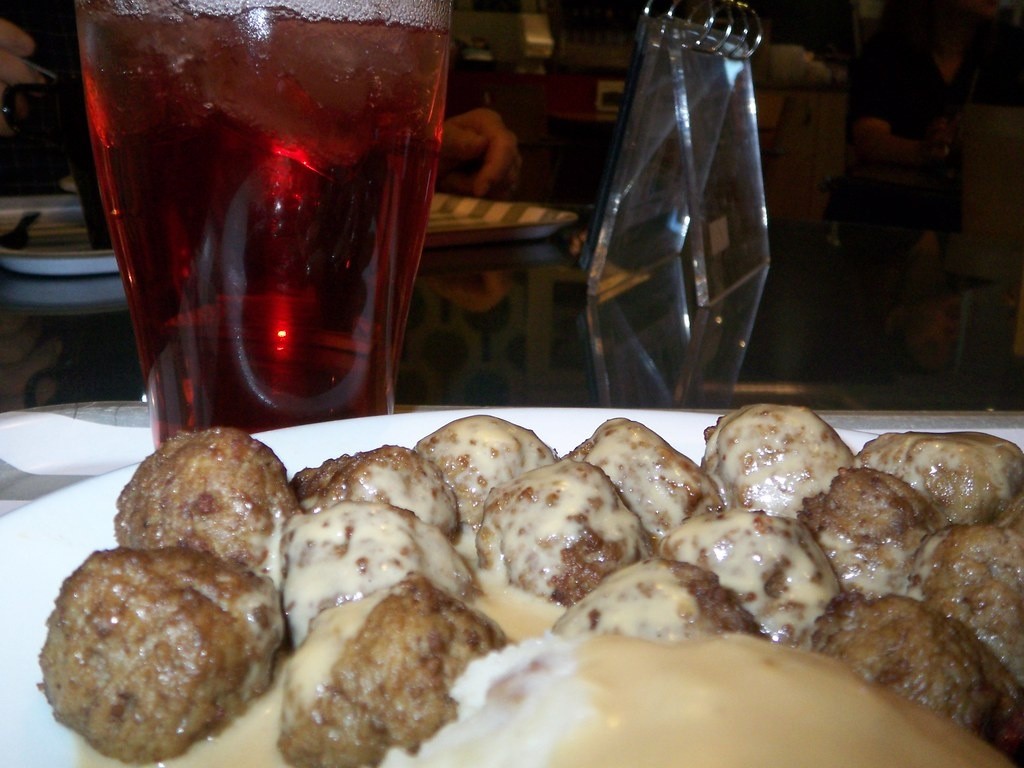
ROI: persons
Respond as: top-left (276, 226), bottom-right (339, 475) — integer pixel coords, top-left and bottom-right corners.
top-left (825, 221), bottom-right (1014, 377)
top-left (822, 0), bottom-right (1024, 233)
top-left (0, 0), bottom-right (523, 199)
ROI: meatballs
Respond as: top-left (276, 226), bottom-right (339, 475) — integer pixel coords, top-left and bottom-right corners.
top-left (38, 403), bottom-right (1023, 768)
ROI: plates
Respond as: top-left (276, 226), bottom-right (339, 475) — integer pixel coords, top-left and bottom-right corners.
top-left (1, 405), bottom-right (878, 768)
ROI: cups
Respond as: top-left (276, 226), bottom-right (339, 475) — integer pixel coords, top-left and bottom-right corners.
top-left (73, 156), bottom-right (113, 252)
top-left (75, 1), bottom-right (449, 454)
